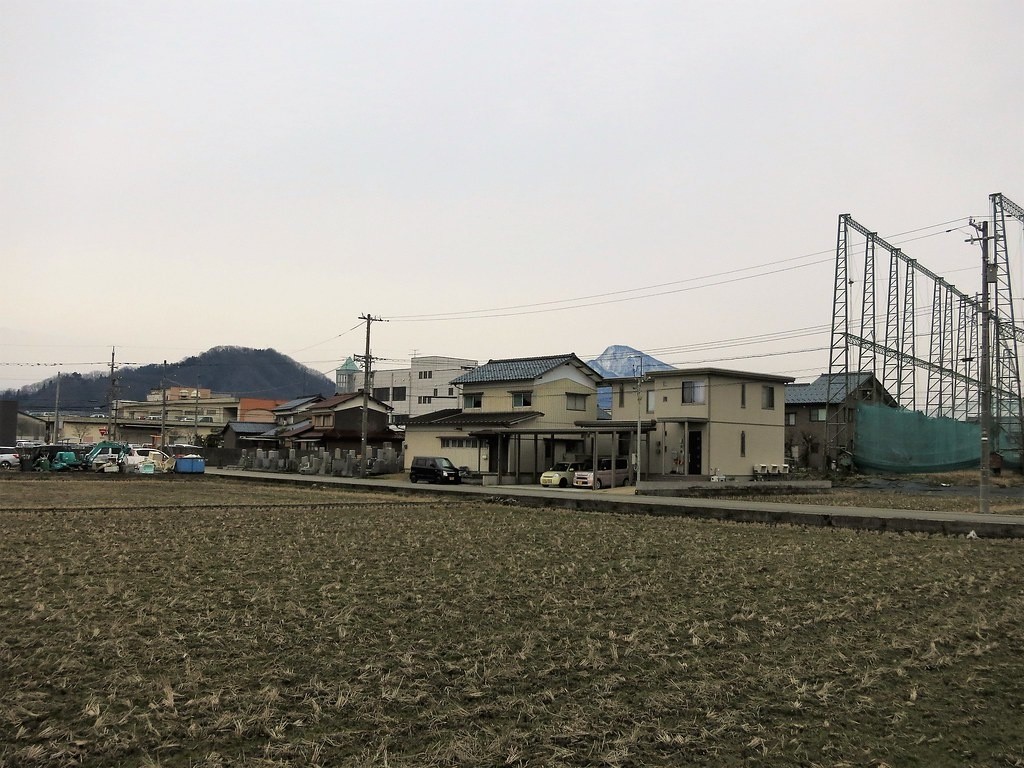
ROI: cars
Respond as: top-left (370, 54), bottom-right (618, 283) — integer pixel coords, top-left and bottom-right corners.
top-left (1, 446), bottom-right (22, 470)
top-left (540, 461), bottom-right (586, 487)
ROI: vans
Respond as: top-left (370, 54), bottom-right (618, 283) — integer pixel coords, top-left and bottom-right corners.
top-left (571, 458), bottom-right (633, 489)
top-left (410, 456), bottom-right (462, 484)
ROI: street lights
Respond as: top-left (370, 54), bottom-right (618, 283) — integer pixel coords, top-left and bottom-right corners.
top-left (627, 354), bottom-right (652, 482)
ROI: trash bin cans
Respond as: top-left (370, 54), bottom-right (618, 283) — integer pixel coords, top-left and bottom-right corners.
top-left (174, 458), bottom-right (205, 473)
